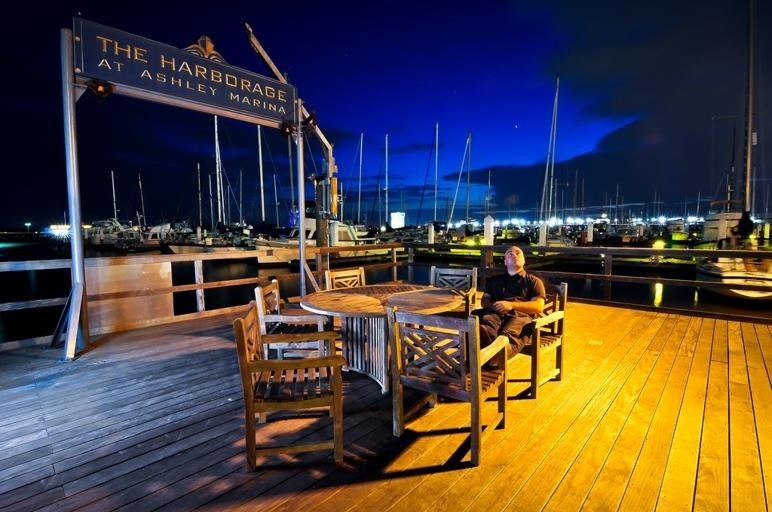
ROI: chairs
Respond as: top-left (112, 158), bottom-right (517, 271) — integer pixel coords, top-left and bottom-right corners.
top-left (386, 304), bottom-right (509, 468)
top-left (519, 281), bottom-right (569, 400)
top-left (234, 300), bottom-right (347, 471)
top-left (429, 266), bottom-right (478, 318)
top-left (324, 267), bottom-right (366, 289)
top-left (255, 280), bottom-right (329, 360)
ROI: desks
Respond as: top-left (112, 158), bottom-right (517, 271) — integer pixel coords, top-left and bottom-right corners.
top-left (300, 284), bottom-right (462, 396)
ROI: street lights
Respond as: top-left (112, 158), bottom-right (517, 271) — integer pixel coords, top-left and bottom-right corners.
top-left (24, 223), bottom-right (32, 232)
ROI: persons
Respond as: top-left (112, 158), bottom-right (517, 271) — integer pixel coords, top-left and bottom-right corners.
top-left (465, 245), bottom-right (547, 367)
